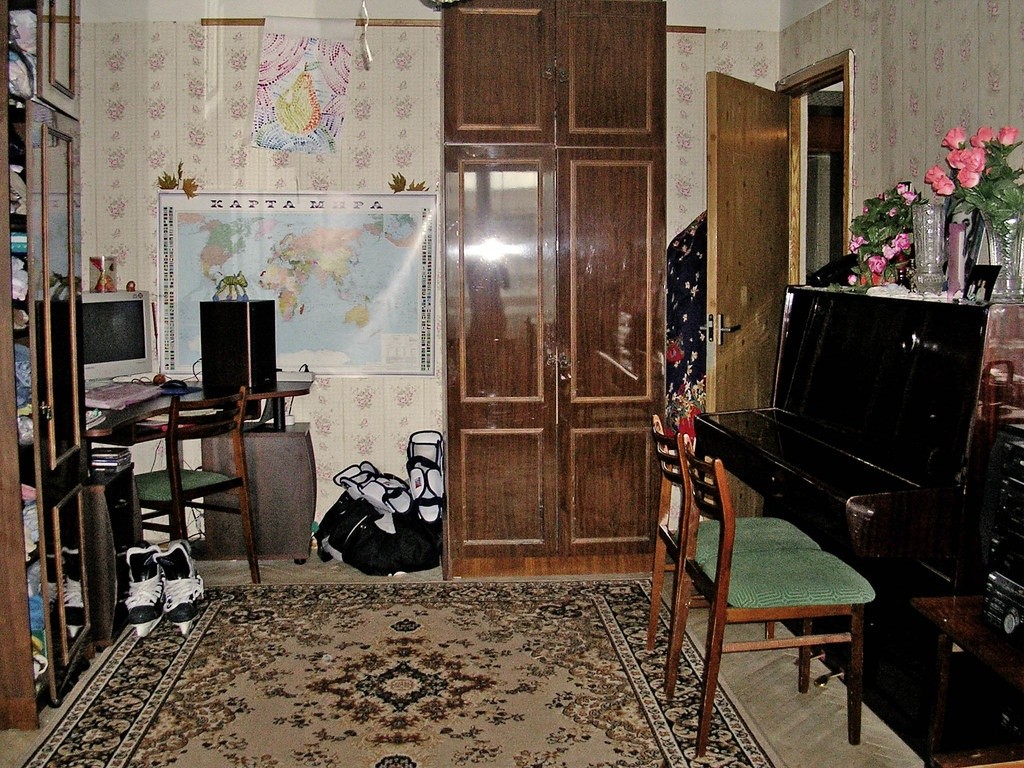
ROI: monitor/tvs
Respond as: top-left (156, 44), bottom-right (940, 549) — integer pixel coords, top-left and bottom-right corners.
top-left (82, 290), bottom-right (153, 390)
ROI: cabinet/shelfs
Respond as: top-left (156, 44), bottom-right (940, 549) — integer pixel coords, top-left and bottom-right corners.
top-left (436, 1), bottom-right (667, 576)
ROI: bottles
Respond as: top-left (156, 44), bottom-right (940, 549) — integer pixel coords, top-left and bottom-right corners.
top-left (894, 268), bottom-right (911, 292)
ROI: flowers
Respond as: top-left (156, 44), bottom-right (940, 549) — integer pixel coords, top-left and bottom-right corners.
top-left (843, 180), bottom-right (923, 294)
top-left (924, 122), bottom-right (1024, 276)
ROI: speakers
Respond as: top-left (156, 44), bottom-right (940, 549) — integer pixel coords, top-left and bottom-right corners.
top-left (200, 300), bottom-right (277, 396)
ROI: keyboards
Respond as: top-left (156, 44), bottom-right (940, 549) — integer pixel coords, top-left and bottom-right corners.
top-left (83, 382), bottom-right (163, 409)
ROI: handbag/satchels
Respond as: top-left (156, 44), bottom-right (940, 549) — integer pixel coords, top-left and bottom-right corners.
top-left (316, 489), bottom-right (442, 577)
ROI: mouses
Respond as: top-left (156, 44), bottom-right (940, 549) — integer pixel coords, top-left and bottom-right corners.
top-left (162, 380), bottom-right (187, 389)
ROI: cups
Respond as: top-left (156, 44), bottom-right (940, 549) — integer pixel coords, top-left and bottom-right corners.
top-left (88, 255), bottom-right (117, 294)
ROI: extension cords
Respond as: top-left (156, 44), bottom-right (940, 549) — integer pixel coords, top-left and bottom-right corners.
top-left (276, 371), bottom-right (315, 381)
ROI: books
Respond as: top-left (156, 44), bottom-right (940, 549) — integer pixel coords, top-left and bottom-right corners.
top-left (91, 448), bottom-right (132, 473)
top-left (86, 409), bottom-right (105, 431)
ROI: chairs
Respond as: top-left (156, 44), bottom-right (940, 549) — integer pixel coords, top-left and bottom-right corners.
top-left (646, 415), bottom-right (877, 759)
top-left (133, 387), bottom-right (260, 586)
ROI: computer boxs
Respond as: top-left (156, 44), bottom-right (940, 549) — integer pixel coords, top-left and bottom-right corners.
top-left (200, 421), bottom-right (318, 560)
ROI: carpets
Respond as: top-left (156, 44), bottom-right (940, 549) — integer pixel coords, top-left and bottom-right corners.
top-left (20, 574), bottom-right (787, 767)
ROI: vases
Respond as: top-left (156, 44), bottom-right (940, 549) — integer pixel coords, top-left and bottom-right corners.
top-left (912, 204), bottom-right (945, 293)
top-left (979, 211), bottom-right (1024, 303)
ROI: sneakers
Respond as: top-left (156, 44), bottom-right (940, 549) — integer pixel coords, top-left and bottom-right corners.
top-left (60, 547), bottom-right (86, 636)
top-left (124, 546), bottom-right (163, 636)
top-left (154, 540), bottom-right (205, 635)
top-left (44, 555), bottom-right (59, 615)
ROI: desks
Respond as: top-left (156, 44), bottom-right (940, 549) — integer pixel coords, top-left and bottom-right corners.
top-left (87, 379), bottom-right (316, 559)
top-left (909, 592), bottom-right (1024, 765)
